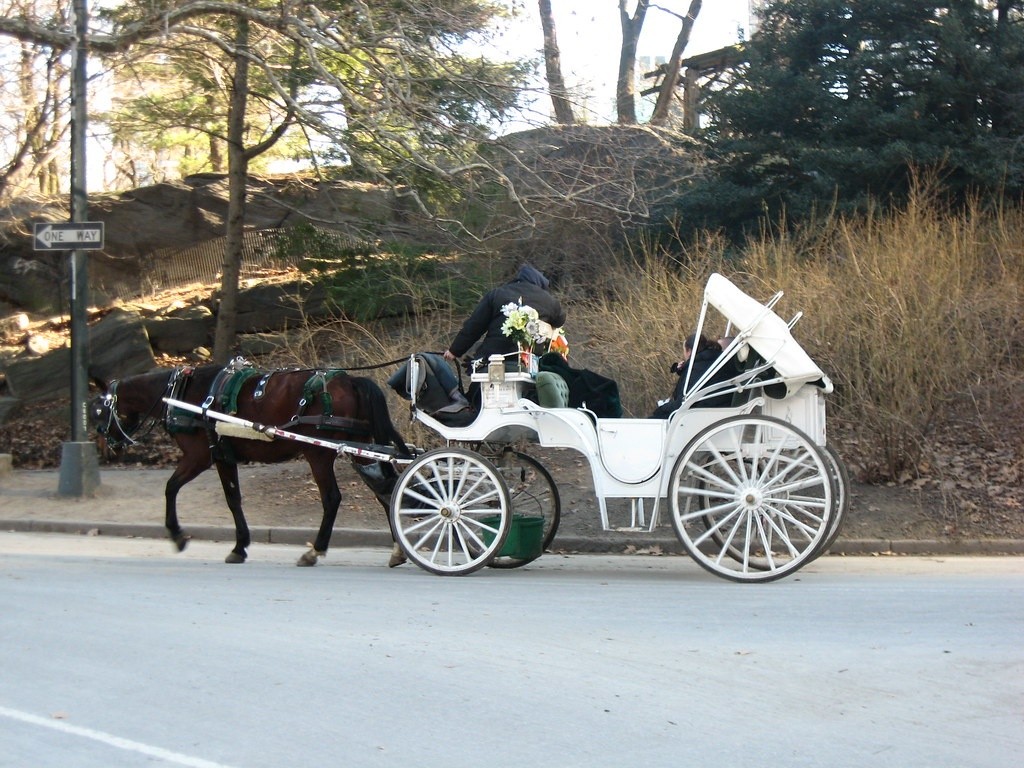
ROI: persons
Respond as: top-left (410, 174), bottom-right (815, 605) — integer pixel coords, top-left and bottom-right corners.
top-left (650, 334), bottom-right (737, 419)
top-left (443, 266), bottom-right (566, 403)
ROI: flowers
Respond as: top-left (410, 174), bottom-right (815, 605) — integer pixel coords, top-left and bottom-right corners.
top-left (500, 295), bottom-right (570, 363)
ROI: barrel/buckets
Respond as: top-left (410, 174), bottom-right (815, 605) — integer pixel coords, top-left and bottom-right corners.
top-left (476, 489), bottom-right (546, 561)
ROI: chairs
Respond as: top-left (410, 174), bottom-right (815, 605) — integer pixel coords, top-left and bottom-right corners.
top-left (471, 352), bottom-right (538, 423)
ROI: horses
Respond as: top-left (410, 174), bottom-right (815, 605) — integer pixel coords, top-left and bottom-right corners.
top-left (98, 360), bottom-right (413, 570)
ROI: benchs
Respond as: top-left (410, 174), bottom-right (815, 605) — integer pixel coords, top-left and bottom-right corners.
top-left (536, 371), bottom-right (595, 448)
top-left (668, 372), bottom-right (756, 452)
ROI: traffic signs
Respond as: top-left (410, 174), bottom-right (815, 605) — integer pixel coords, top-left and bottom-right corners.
top-left (33, 221), bottom-right (106, 251)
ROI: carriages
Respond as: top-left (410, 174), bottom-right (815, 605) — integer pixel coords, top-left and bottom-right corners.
top-left (85, 272), bottom-right (849, 576)
